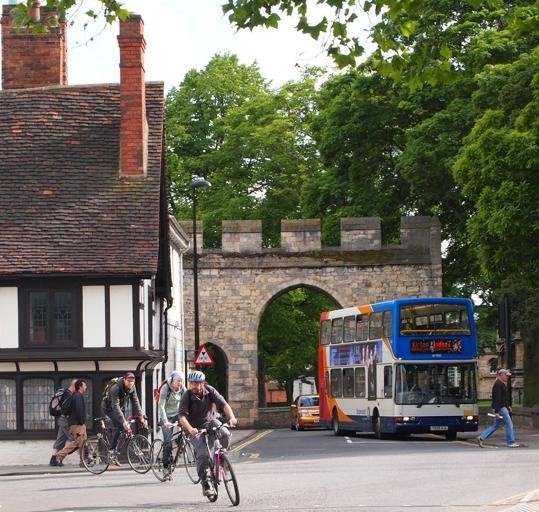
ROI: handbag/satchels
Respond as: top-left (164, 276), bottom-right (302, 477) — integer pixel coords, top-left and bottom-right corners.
top-left (103, 377), bottom-right (121, 400)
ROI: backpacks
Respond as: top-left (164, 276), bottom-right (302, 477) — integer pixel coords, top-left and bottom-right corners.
top-left (48, 388), bottom-right (65, 416)
top-left (156, 381), bottom-right (184, 407)
top-left (62, 392), bottom-right (77, 414)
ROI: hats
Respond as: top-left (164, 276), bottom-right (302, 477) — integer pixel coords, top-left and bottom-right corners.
top-left (172, 372), bottom-right (182, 379)
top-left (499, 369), bottom-right (511, 375)
top-left (125, 373), bottom-right (135, 380)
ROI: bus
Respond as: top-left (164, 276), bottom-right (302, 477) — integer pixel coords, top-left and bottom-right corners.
top-left (318, 294), bottom-right (480, 443)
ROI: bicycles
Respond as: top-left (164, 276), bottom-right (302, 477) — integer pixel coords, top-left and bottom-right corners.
top-left (193, 420), bottom-right (241, 506)
top-left (148, 421), bottom-right (201, 484)
top-left (80, 417), bottom-right (153, 474)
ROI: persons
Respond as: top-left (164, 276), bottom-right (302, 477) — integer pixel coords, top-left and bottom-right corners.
top-left (178, 371), bottom-right (237, 496)
top-left (50, 381), bottom-right (93, 468)
top-left (477, 369), bottom-right (520, 448)
top-left (101, 372), bottom-right (149, 466)
top-left (49, 378), bottom-right (83, 465)
top-left (158, 370), bottom-right (188, 481)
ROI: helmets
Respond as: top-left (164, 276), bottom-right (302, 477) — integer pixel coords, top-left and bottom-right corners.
top-left (187, 371), bottom-right (205, 382)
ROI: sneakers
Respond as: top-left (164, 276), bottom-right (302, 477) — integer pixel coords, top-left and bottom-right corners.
top-left (508, 444), bottom-right (519, 448)
top-left (51, 456), bottom-right (64, 466)
top-left (478, 438), bottom-right (484, 447)
top-left (202, 480), bottom-right (217, 496)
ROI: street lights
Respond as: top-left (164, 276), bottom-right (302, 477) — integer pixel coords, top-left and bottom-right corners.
top-left (191, 180), bottom-right (214, 370)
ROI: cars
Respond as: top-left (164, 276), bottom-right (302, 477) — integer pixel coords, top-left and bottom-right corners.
top-left (290, 394), bottom-right (321, 430)
